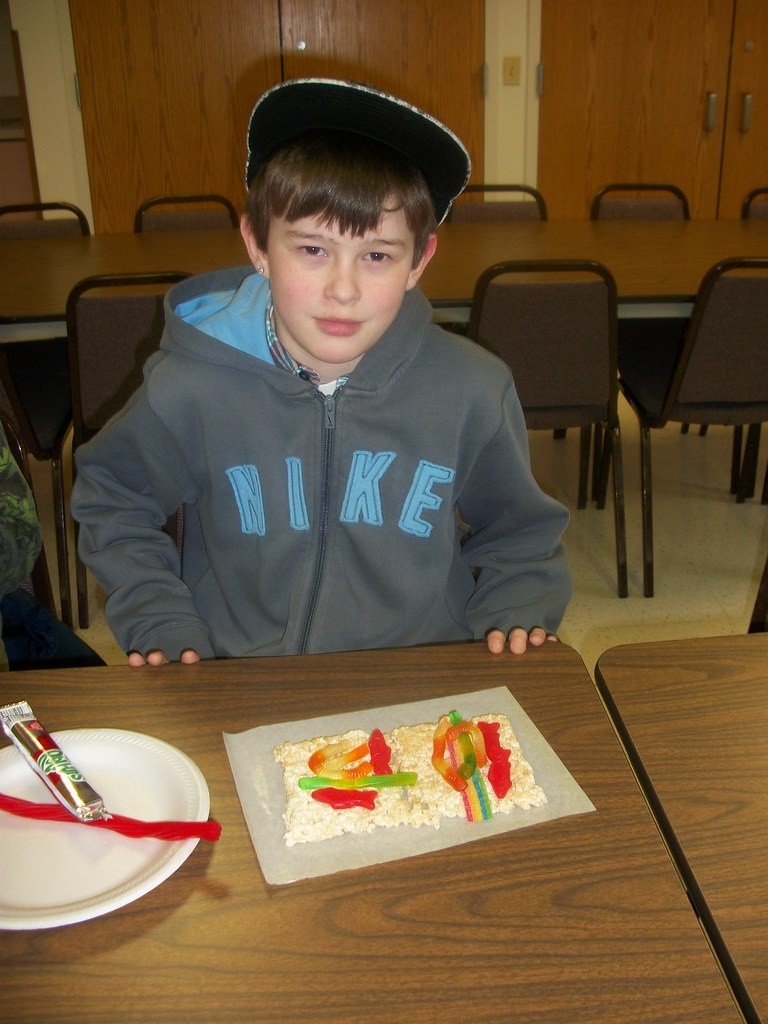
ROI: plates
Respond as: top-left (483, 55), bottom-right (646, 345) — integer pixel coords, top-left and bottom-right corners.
top-left (0, 727), bottom-right (210, 933)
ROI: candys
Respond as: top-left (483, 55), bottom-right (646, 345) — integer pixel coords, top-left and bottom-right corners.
top-left (298, 710), bottom-right (510, 823)
top-left (0, 793), bottom-right (222, 842)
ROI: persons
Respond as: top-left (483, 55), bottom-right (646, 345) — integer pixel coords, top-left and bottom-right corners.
top-left (67, 80), bottom-right (573, 667)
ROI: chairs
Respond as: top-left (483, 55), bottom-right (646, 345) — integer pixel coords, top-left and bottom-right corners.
top-left (0, 202), bottom-right (91, 239)
top-left (134, 193), bottom-right (241, 233)
top-left (66, 270), bottom-right (195, 629)
top-left (445, 183), bottom-right (548, 222)
top-left (741, 187), bottom-right (768, 222)
top-left (466, 258), bottom-right (629, 598)
top-left (587, 183), bottom-right (690, 220)
top-left (0, 336), bottom-right (75, 629)
top-left (595, 254), bottom-right (768, 598)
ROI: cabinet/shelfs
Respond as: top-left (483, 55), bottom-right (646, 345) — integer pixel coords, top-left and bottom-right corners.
top-left (68, 1), bottom-right (487, 230)
top-left (529, 0), bottom-right (768, 222)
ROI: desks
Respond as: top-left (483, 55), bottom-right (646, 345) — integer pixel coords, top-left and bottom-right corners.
top-left (0, 218), bottom-right (768, 512)
top-left (0, 642), bottom-right (747, 1022)
top-left (595, 631), bottom-right (768, 1024)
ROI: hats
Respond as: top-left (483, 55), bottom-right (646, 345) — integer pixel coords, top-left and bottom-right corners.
top-left (245, 78), bottom-right (472, 229)
